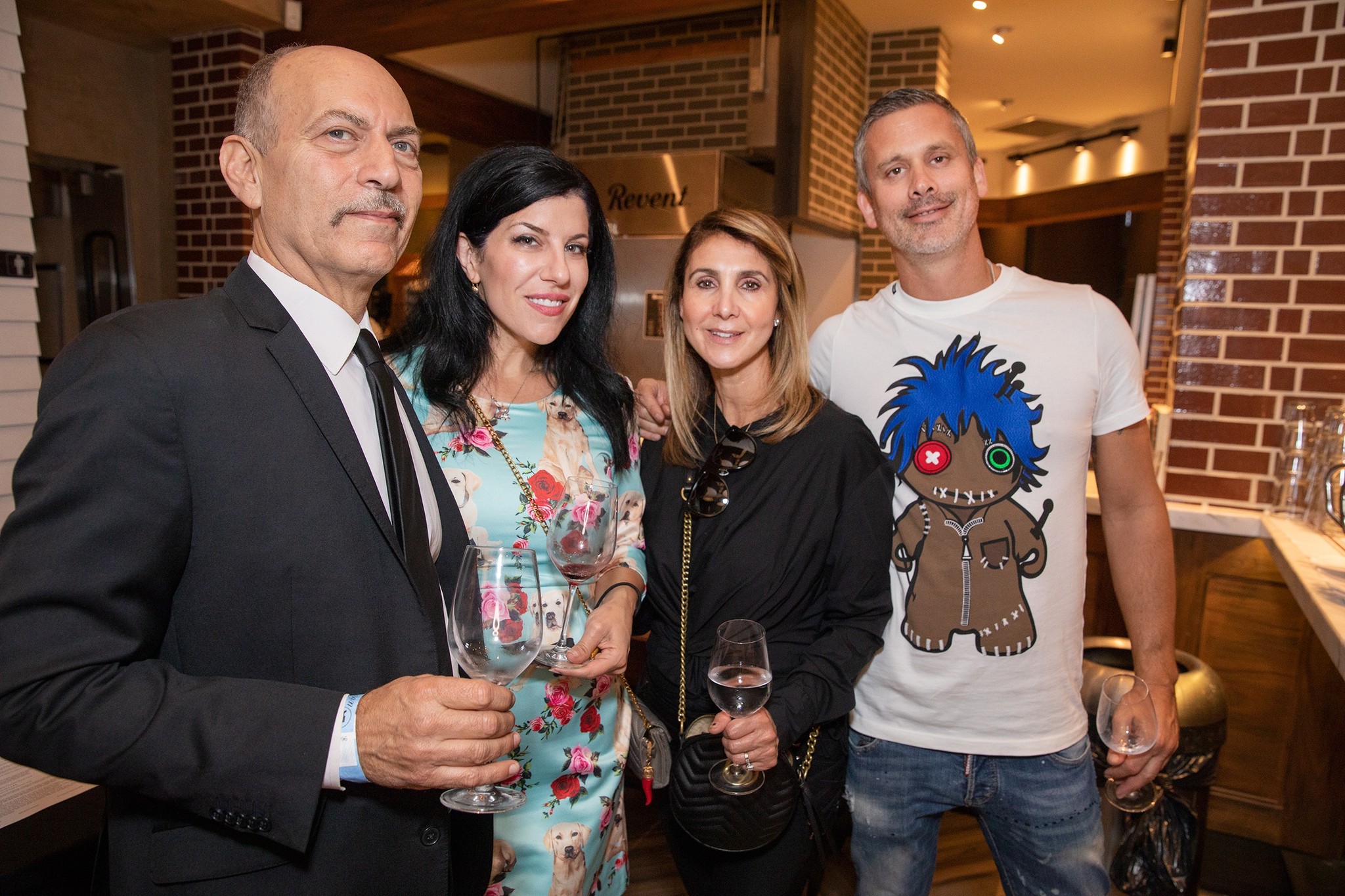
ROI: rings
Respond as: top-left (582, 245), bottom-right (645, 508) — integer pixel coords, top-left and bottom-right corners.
top-left (744, 751), bottom-right (754, 770)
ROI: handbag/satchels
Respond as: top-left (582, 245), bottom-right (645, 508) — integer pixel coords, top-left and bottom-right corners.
top-left (669, 714), bottom-right (799, 853)
top-left (628, 689), bottom-right (672, 790)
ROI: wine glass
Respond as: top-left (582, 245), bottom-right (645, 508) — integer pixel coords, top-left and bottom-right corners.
top-left (707, 619), bottom-right (773, 796)
top-left (529, 476), bottom-right (618, 669)
top-left (439, 545), bottom-right (544, 811)
top-left (1096, 674), bottom-right (1159, 812)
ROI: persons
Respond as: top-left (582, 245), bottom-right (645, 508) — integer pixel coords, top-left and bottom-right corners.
top-left (633, 86), bottom-right (1178, 896)
top-left (377, 145), bottom-right (647, 896)
top-left (622, 210), bottom-right (894, 896)
top-left (0, 44), bottom-right (520, 896)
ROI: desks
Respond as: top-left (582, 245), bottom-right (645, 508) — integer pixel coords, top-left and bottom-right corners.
top-left (1084, 467), bottom-right (1345, 896)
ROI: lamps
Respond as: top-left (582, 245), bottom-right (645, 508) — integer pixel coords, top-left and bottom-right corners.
top-left (991, 25), bottom-right (1015, 45)
top-left (970, 0), bottom-right (988, 10)
top-left (1007, 124), bottom-right (1140, 167)
top-left (1160, 36), bottom-right (1178, 58)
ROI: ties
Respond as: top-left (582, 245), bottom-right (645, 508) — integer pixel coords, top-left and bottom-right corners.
top-left (353, 328), bottom-right (454, 676)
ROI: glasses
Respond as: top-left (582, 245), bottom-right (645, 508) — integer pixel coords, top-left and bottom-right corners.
top-left (687, 425), bottom-right (759, 518)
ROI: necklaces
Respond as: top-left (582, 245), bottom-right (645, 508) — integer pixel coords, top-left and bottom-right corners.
top-left (471, 355), bottom-right (541, 420)
top-left (985, 257), bottom-right (996, 285)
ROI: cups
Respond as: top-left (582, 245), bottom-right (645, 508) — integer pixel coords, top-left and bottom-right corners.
top-left (1268, 398), bottom-right (1345, 531)
